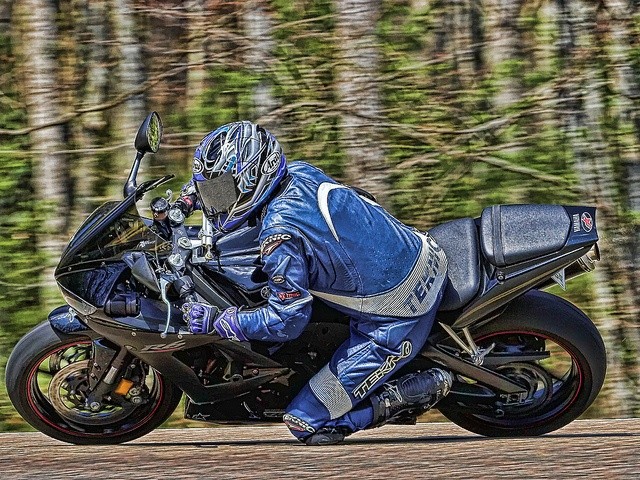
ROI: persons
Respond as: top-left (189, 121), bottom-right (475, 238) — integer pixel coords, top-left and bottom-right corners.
top-left (181, 121), bottom-right (453, 445)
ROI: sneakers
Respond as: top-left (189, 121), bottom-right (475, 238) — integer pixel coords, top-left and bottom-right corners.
top-left (372, 366), bottom-right (452, 428)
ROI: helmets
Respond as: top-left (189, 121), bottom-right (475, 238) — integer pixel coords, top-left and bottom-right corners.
top-left (192, 121), bottom-right (287, 233)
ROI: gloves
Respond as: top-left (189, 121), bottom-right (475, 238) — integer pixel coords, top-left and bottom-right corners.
top-left (181, 181), bottom-right (202, 209)
top-left (182, 301), bottom-right (218, 334)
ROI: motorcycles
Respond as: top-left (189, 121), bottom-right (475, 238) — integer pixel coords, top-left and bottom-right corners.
top-left (5, 110), bottom-right (607, 446)
